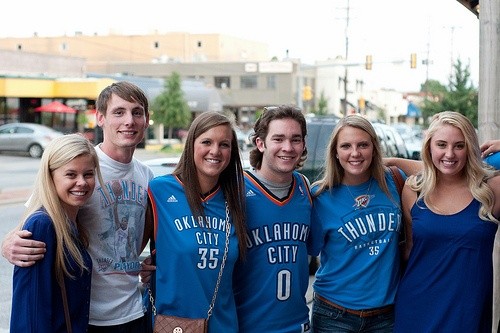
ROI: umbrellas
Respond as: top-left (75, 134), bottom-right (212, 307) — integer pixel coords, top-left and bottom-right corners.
top-left (35, 102), bottom-right (78, 128)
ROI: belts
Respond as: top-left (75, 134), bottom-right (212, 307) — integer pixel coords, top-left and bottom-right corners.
top-left (315, 294), bottom-right (390, 317)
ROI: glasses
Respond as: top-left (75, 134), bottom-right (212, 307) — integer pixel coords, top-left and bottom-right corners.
top-left (260, 105), bottom-right (301, 120)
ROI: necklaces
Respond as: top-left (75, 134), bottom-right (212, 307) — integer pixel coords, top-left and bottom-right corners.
top-left (342, 176), bottom-right (372, 207)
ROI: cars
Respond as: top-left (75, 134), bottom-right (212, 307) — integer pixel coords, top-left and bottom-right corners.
top-left (0, 122), bottom-right (65, 159)
top-left (295, 113), bottom-right (426, 188)
top-left (235, 132), bottom-right (248, 151)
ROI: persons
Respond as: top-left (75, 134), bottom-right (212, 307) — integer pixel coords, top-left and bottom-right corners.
top-left (2, 82), bottom-right (152, 333)
top-left (141, 112), bottom-right (248, 333)
top-left (143, 103), bottom-right (314, 333)
top-left (10, 135), bottom-right (111, 333)
top-left (309, 112), bottom-right (500, 333)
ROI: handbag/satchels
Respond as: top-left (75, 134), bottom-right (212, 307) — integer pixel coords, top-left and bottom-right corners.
top-left (153, 314), bottom-right (207, 332)
